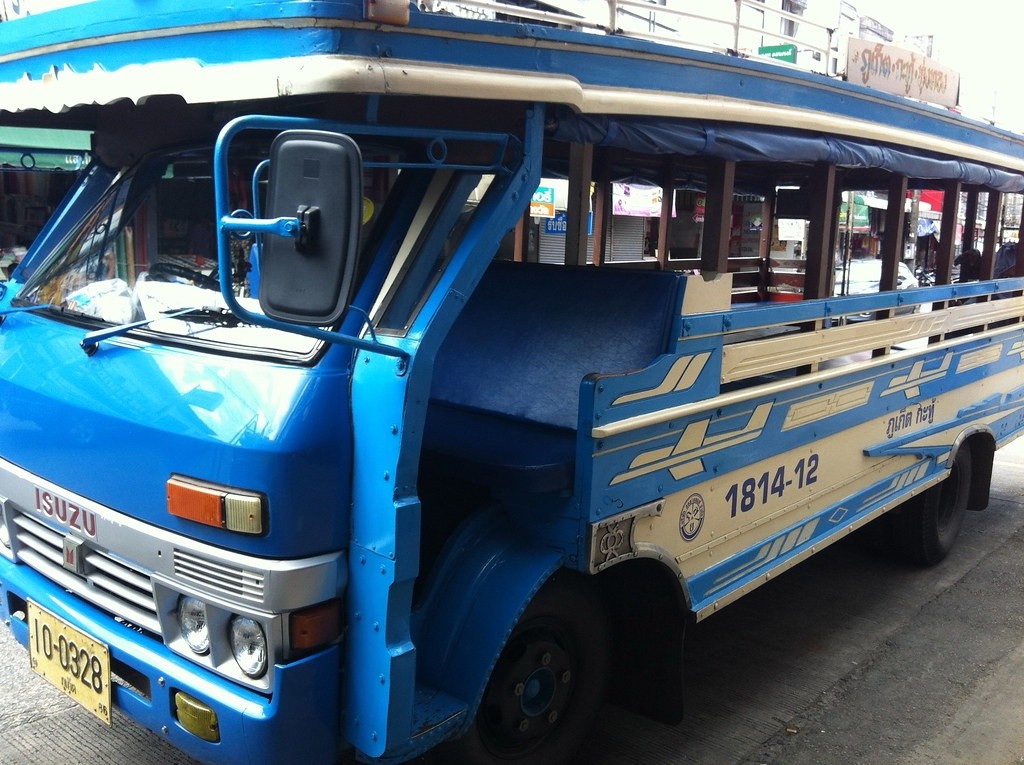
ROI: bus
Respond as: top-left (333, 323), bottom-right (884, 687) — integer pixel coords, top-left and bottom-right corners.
top-left (0, 0), bottom-right (1023, 765)
